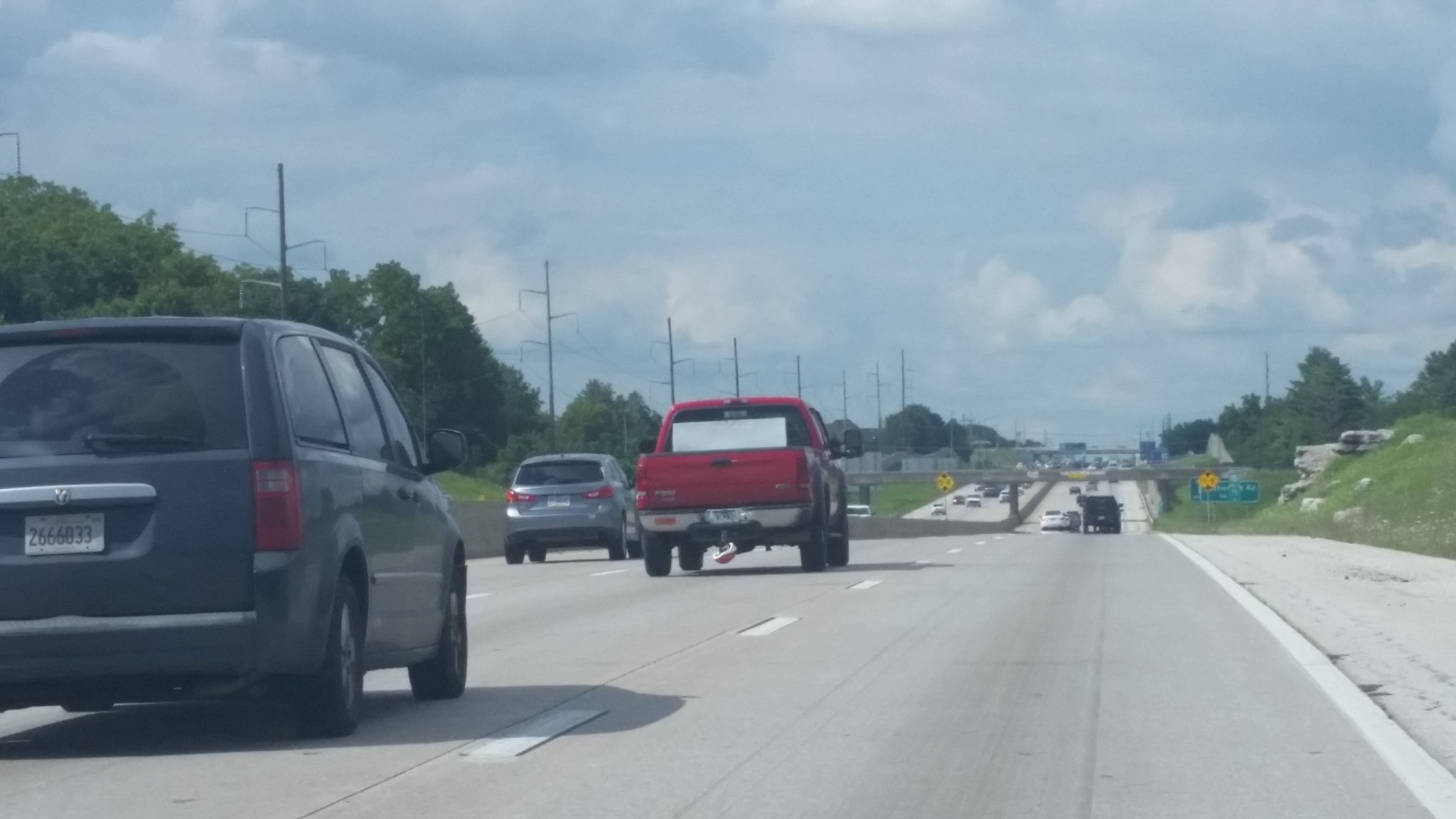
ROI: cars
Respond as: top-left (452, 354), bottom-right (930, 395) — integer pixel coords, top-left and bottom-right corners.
top-left (847, 505), bottom-right (876, 518)
top-left (1038, 509), bottom-right (1066, 530)
top-left (966, 494), bottom-right (982, 508)
top-left (931, 503), bottom-right (945, 515)
top-left (502, 453), bottom-right (644, 565)
top-left (953, 496), bottom-right (966, 505)
top-left (1068, 485), bottom-right (1083, 496)
top-left (2, 313), bottom-right (471, 743)
top-left (973, 457), bottom-right (1135, 534)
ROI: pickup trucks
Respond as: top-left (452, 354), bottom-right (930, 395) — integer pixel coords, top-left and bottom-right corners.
top-left (635, 396), bottom-right (866, 577)
